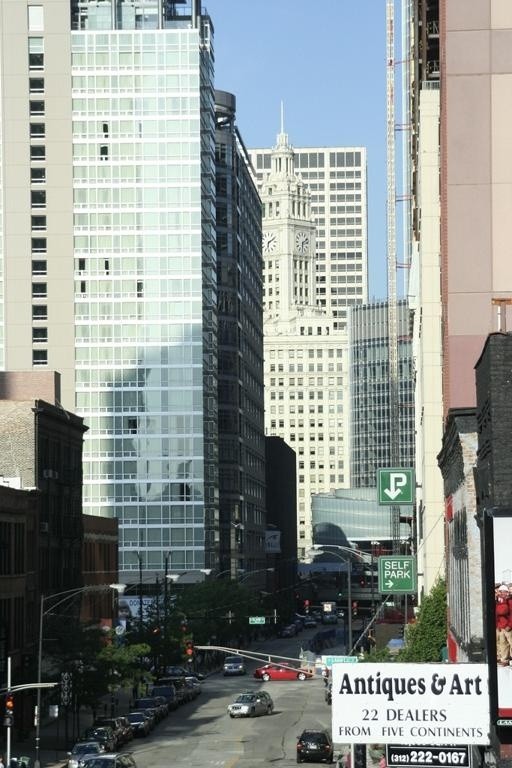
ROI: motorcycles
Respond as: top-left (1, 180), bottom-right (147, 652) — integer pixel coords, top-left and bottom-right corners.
top-left (322, 671), bottom-right (329, 684)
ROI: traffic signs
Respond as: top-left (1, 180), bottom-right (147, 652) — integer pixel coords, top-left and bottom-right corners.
top-left (249, 617), bottom-right (265, 624)
top-left (322, 655), bottom-right (358, 664)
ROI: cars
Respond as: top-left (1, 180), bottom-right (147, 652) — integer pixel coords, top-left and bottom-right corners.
top-left (325, 682), bottom-right (332, 704)
top-left (253, 662), bottom-right (312, 681)
top-left (66, 674), bottom-right (204, 768)
top-left (279, 610), bottom-right (346, 638)
top-left (385, 638), bottom-right (404, 657)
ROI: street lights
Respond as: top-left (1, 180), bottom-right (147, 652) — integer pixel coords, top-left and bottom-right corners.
top-left (309, 549), bottom-right (352, 652)
top-left (245, 565), bottom-right (278, 578)
top-left (312, 543), bottom-right (380, 567)
top-left (155, 568), bottom-right (213, 660)
top-left (34, 582), bottom-right (127, 768)
top-left (216, 566), bottom-right (243, 577)
top-left (110, 572), bottom-right (184, 646)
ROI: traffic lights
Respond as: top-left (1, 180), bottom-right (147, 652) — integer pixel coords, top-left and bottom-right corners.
top-left (181, 625), bottom-right (187, 632)
top-left (398, 627), bottom-right (404, 638)
top-left (371, 630), bottom-right (375, 636)
top-left (304, 600), bottom-right (310, 614)
top-left (360, 575), bottom-right (365, 585)
top-left (353, 601), bottom-right (358, 615)
top-left (5, 692), bottom-right (15, 714)
top-left (185, 639), bottom-right (194, 662)
top-left (5, 716), bottom-right (13, 726)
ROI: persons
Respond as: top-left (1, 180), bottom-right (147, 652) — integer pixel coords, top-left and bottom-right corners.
top-left (238, 627), bottom-right (274, 646)
top-left (196, 652), bottom-right (222, 672)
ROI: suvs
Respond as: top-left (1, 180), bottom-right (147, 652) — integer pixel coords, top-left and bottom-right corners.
top-left (295, 727), bottom-right (332, 763)
top-left (227, 690), bottom-right (274, 719)
top-left (223, 655), bottom-right (246, 675)
top-left (150, 665), bottom-right (208, 682)
